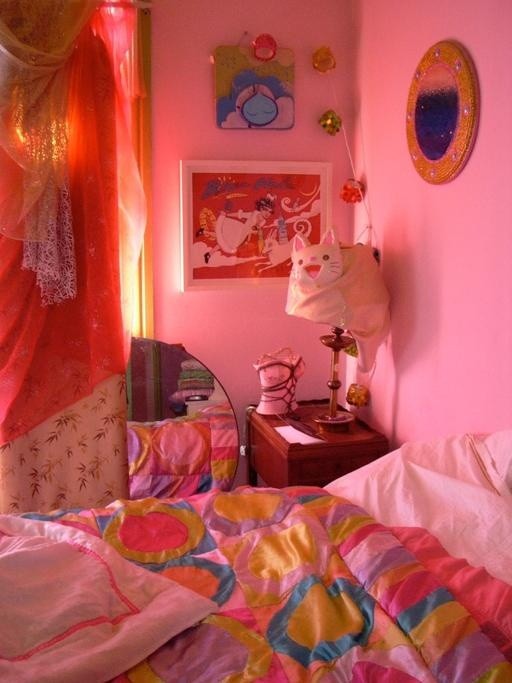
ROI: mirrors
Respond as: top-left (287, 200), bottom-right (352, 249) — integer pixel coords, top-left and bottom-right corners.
top-left (406, 39), bottom-right (480, 184)
top-left (126, 337), bottom-right (241, 500)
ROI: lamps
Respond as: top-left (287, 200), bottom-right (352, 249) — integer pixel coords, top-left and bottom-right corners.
top-left (284, 227), bottom-right (393, 429)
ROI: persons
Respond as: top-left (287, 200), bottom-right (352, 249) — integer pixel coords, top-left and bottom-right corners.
top-left (195, 198), bottom-right (274, 265)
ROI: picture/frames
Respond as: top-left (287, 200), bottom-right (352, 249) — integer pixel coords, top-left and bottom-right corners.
top-left (179, 158), bottom-right (334, 292)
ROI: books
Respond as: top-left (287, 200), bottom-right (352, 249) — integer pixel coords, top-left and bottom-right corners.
top-left (273, 410), bottom-right (326, 444)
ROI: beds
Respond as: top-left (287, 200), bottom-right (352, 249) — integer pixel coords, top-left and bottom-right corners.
top-left (0, 429), bottom-right (512, 683)
top-left (126, 401), bottom-right (239, 500)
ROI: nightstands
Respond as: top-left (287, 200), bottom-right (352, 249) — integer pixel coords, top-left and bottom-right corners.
top-left (245, 399), bottom-right (388, 487)
